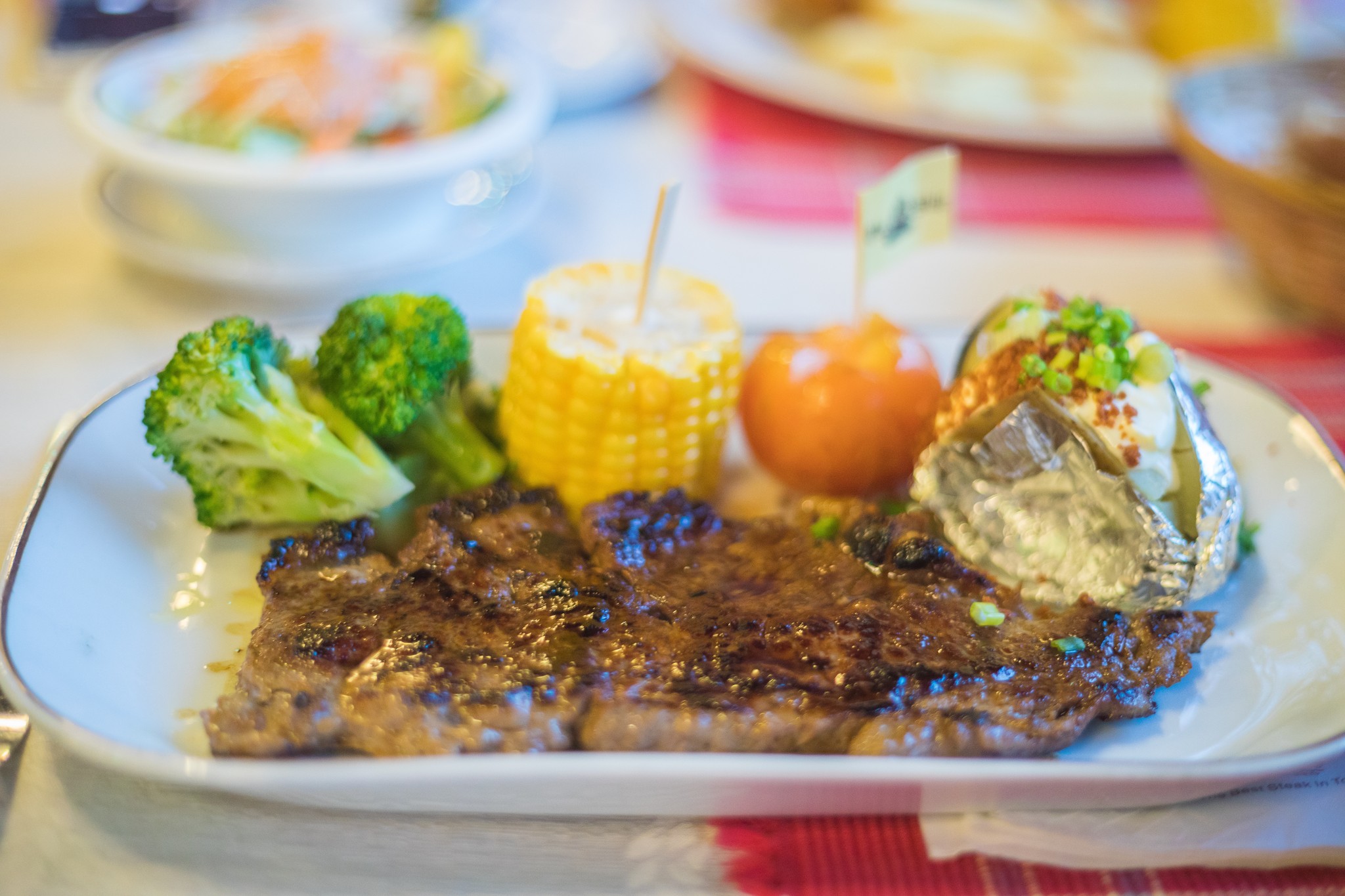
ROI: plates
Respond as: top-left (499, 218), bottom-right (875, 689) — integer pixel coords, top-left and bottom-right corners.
top-left (632, 2), bottom-right (1286, 159)
top-left (3, 330), bottom-right (1345, 817)
top-left (84, 158), bottom-right (558, 301)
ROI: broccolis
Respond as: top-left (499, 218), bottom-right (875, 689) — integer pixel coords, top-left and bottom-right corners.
top-left (140, 288), bottom-right (506, 521)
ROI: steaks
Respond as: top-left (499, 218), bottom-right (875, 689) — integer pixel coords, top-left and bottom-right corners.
top-left (202, 480), bottom-right (1222, 755)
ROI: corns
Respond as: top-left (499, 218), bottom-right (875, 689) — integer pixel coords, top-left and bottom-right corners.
top-left (495, 255), bottom-right (746, 515)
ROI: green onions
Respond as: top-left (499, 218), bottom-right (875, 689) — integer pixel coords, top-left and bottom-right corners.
top-left (1019, 294), bottom-right (1172, 389)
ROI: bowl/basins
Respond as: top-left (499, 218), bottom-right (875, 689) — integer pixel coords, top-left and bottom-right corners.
top-left (1159, 55), bottom-right (1345, 335)
top-left (73, 11), bottom-right (559, 273)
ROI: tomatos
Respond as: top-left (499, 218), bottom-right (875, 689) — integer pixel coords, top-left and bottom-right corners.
top-left (740, 313), bottom-right (948, 498)
